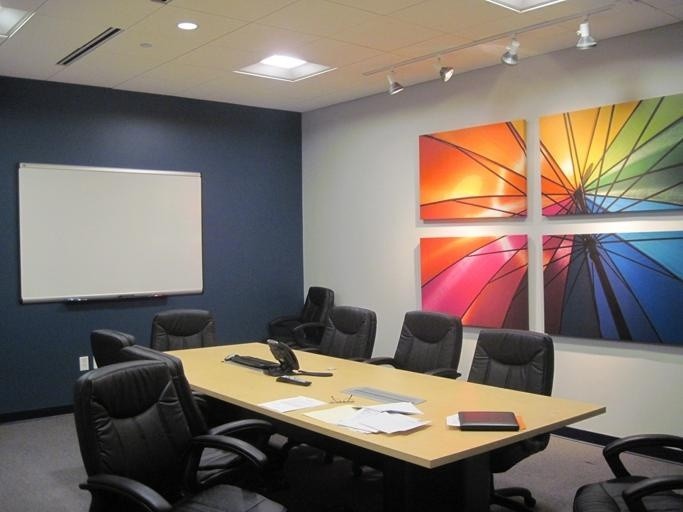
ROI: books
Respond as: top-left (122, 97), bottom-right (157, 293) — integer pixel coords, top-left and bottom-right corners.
top-left (447, 411), bottom-right (525, 429)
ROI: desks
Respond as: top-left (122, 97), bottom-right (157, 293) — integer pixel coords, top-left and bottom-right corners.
top-left (156, 342), bottom-right (605, 512)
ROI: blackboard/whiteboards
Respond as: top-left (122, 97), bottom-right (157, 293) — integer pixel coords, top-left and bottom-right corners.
top-left (18, 162), bottom-right (205, 303)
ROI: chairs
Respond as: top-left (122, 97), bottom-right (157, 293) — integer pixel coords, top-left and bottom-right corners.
top-left (74, 359), bottom-right (286, 512)
top-left (464, 328), bottom-right (556, 512)
top-left (121, 348), bottom-right (287, 488)
top-left (151, 312), bottom-right (216, 352)
top-left (272, 286), bottom-right (336, 341)
top-left (303, 307), bottom-right (377, 365)
top-left (90, 328), bottom-right (210, 418)
top-left (573, 431), bottom-right (681, 512)
top-left (366, 310), bottom-right (466, 379)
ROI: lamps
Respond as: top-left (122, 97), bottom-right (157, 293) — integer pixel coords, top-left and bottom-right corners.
top-left (382, 10), bottom-right (597, 95)
